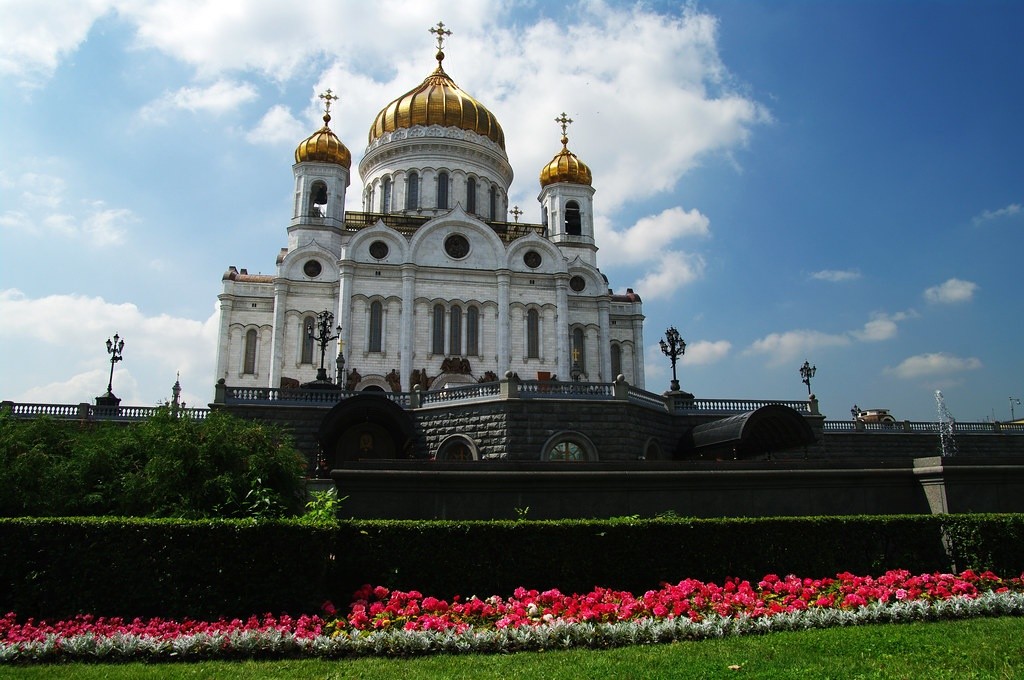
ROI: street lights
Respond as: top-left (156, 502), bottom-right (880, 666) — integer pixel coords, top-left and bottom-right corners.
top-left (94, 332), bottom-right (126, 406)
top-left (658, 325), bottom-right (686, 389)
top-left (799, 359), bottom-right (816, 394)
top-left (305, 308), bottom-right (345, 390)
top-left (166, 372), bottom-right (188, 408)
top-left (1007, 395), bottom-right (1023, 421)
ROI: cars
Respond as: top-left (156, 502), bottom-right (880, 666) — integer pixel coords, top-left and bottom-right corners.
top-left (857, 408), bottom-right (898, 422)
